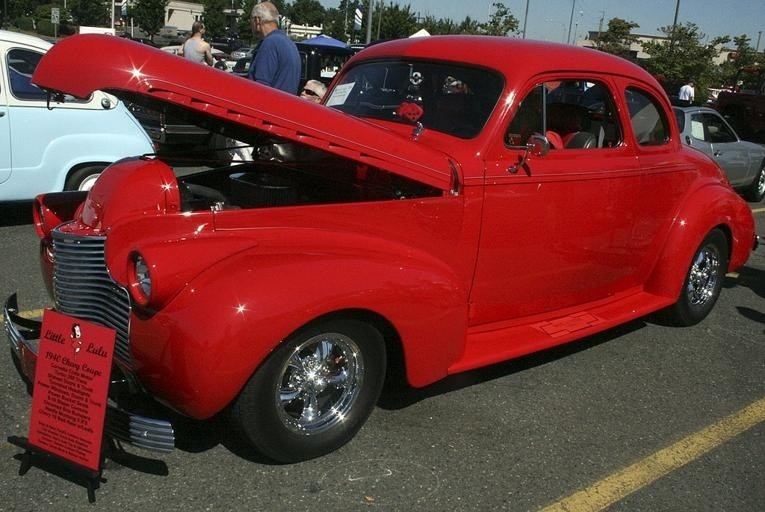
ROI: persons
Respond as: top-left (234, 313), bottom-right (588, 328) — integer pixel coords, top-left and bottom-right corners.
top-left (181, 21), bottom-right (213, 70)
top-left (678, 80), bottom-right (695, 106)
top-left (229, 0), bottom-right (303, 169)
top-left (299, 79), bottom-right (329, 107)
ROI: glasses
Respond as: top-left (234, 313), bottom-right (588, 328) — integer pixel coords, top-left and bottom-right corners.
top-left (302, 88), bottom-right (322, 101)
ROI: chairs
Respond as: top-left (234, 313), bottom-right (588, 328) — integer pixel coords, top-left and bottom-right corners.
top-left (525, 101), bottom-right (598, 153)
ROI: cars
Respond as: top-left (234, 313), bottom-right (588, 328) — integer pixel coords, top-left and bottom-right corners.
top-left (670, 105), bottom-right (765, 203)
top-left (1, 35), bottom-right (760, 469)
top-left (1, 30), bottom-right (160, 215)
top-left (112, 33), bottom-right (366, 168)
